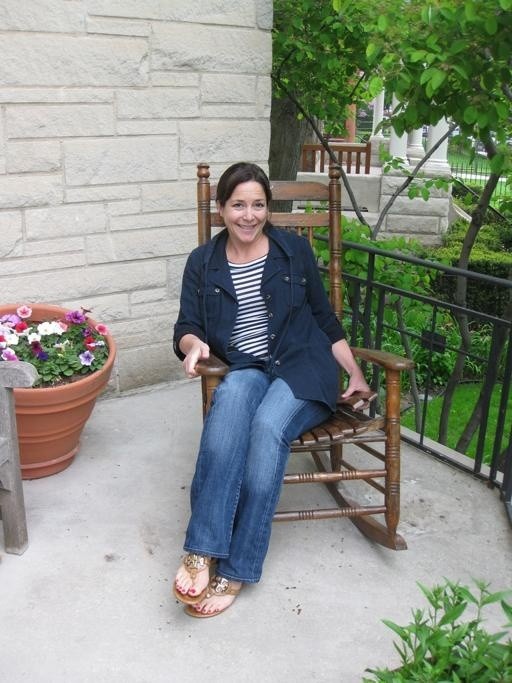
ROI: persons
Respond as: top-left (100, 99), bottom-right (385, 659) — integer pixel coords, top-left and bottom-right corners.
top-left (173, 162), bottom-right (373, 619)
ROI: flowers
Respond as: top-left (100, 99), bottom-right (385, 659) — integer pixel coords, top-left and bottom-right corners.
top-left (0, 305), bottom-right (109, 383)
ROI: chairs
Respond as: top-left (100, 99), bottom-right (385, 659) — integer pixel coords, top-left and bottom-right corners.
top-left (185, 156), bottom-right (417, 553)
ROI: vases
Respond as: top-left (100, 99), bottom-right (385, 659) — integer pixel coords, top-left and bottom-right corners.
top-left (0, 301), bottom-right (118, 480)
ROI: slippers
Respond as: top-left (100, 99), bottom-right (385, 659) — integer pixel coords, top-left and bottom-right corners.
top-left (182, 575), bottom-right (245, 618)
top-left (173, 551), bottom-right (219, 605)
